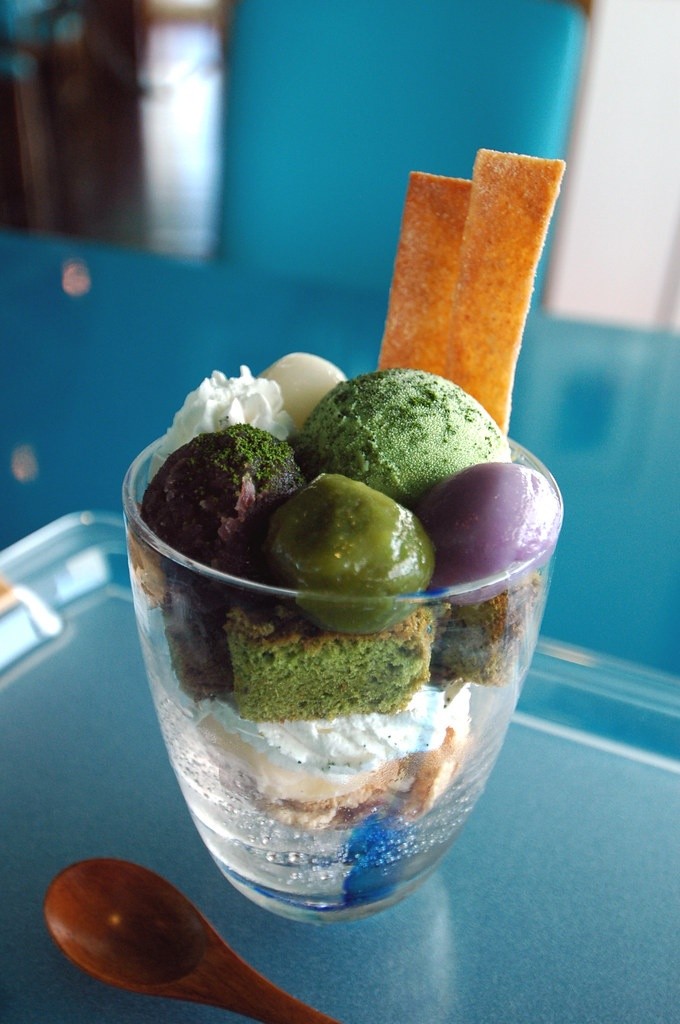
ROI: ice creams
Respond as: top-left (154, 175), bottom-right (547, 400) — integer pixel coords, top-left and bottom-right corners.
top-left (122, 148), bottom-right (564, 922)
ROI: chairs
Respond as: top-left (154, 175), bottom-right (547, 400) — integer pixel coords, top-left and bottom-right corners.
top-left (217, 0), bottom-right (586, 307)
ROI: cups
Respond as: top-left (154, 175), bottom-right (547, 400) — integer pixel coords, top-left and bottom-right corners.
top-left (121, 435), bottom-right (563, 922)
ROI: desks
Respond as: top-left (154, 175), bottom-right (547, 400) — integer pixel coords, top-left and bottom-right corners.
top-left (0, 232), bottom-right (680, 1024)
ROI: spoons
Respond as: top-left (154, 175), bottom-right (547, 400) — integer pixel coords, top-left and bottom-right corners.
top-left (44, 859), bottom-right (338, 1024)
